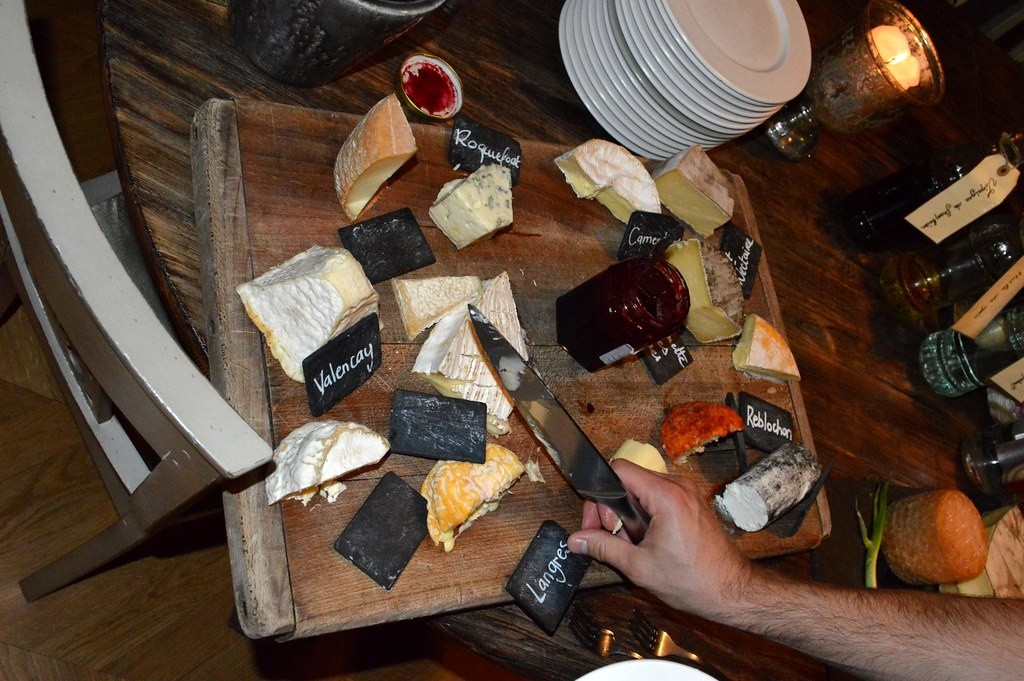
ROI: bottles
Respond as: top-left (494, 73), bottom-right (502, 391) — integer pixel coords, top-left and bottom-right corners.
top-left (919, 306), bottom-right (1023, 396)
top-left (845, 130), bottom-right (1024, 246)
top-left (960, 418), bottom-right (1024, 492)
top-left (882, 219), bottom-right (1024, 327)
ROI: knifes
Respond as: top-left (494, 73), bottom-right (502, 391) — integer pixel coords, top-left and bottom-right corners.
top-left (469, 302), bottom-right (650, 544)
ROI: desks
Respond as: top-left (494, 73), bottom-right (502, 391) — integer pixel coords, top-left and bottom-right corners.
top-left (94, 1), bottom-right (1023, 681)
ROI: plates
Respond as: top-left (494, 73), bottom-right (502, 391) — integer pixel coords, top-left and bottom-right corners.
top-left (558, 0), bottom-right (813, 161)
top-left (574, 658), bottom-right (718, 681)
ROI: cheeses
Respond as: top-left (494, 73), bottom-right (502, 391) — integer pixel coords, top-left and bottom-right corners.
top-left (233, 93), bottom-right (822, 550)
top-left (937, 506), bottom-right (1024, 598)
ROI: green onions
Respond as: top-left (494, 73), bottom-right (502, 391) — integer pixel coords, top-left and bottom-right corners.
top-left (854, 469), bottom-right (889, 588)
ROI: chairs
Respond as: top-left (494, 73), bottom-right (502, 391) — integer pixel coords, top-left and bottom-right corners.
top-left (0, 0), bottom-right (275, 603)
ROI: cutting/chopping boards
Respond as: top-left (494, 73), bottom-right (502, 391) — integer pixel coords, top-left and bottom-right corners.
top-left (191, 97), bottom-right (832, 645)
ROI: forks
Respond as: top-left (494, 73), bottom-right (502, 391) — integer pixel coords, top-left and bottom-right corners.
top-left (628, 605), bottom-right (733, 681)
top-left (568, 602), bottom-right (644, 660)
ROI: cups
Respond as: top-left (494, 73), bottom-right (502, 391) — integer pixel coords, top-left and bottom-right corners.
top-left (228, 0), bottom-right (447, 89)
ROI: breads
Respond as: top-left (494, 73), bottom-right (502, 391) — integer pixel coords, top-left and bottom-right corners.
top-left (878, 489), bottom-right (989, 585)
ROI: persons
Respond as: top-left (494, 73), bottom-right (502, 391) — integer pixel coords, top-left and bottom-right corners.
top-left (568, 457), bottom-right (1024, 681)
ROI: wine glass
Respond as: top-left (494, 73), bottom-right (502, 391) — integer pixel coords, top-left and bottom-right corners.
top-left (766, 0), bottom-right (947, 160)
